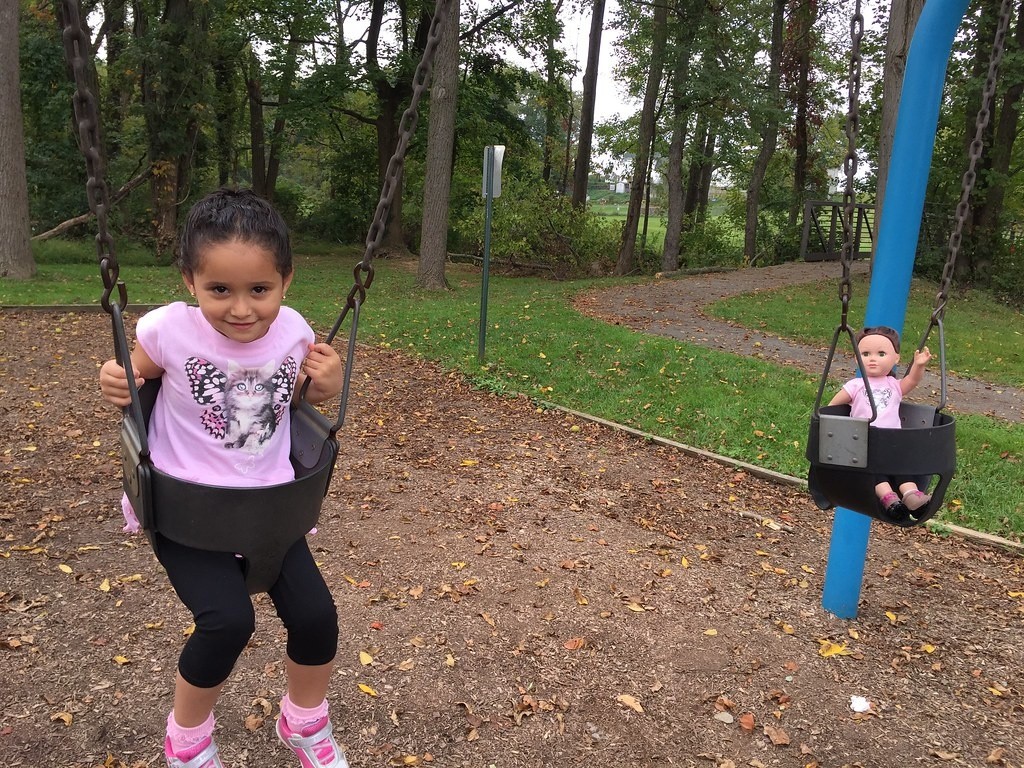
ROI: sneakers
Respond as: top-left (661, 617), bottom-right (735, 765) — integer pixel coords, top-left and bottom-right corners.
top-left (276, 712), bottom-right (349, 768)
top-left (164, 733), bottom-right (226, 768)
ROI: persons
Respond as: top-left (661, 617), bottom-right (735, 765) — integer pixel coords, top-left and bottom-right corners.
top-left (99, 187), bottom-right (351, 768)
top-left (826, 326), bottom-right (932, 523)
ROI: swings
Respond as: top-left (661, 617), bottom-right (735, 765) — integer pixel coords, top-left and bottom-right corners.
top-left (793, 0), bottom-right (1017, 535)
top-left (55, 0), bottom-right (458, 598)
